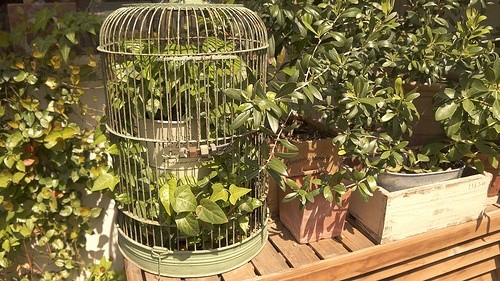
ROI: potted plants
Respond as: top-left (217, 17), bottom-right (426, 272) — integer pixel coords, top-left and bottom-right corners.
top-left (97, 0), bottom-right (500, 279)
